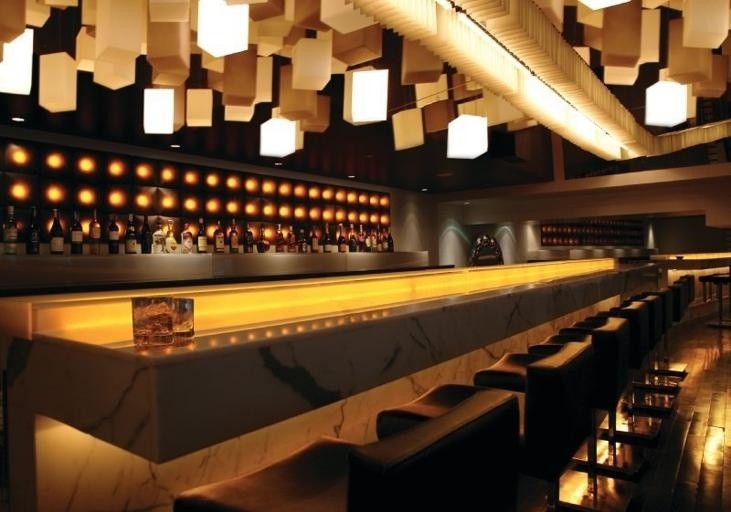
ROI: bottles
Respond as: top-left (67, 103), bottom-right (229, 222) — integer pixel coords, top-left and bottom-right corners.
top-left (4, 205), bottom-right (395, 254)
